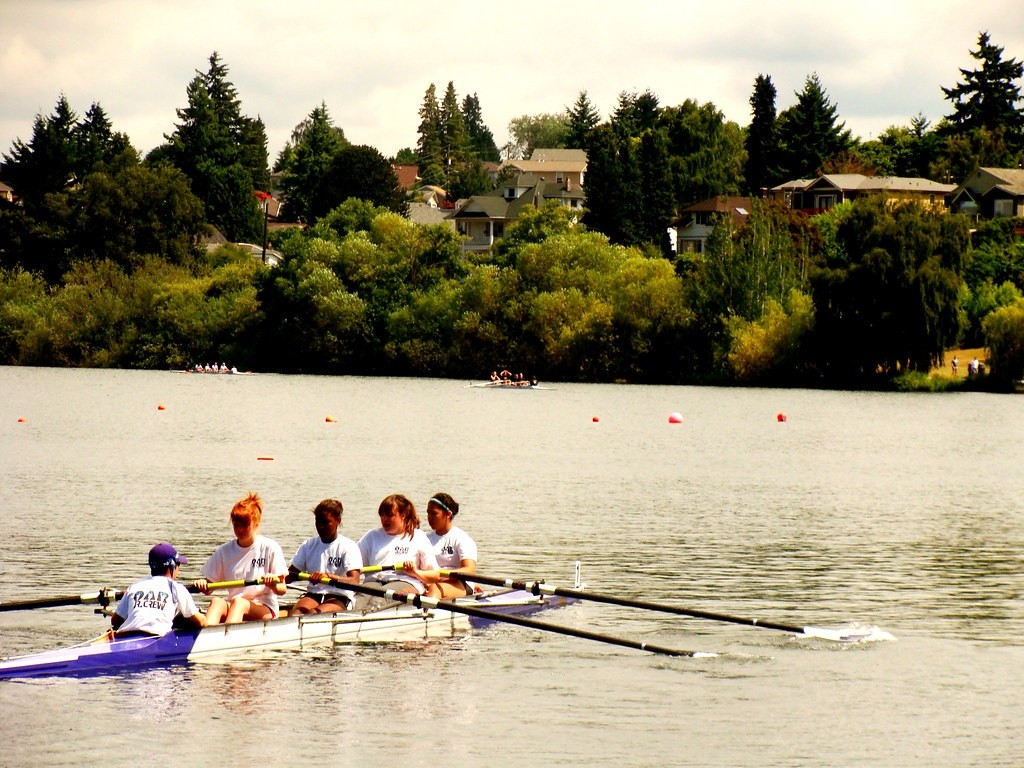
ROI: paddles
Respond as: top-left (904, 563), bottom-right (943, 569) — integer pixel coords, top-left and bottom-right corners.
top-left (358, 563), bottom-right (405, 573)
top-left (440, 569), bottom-right (874, 643)
top-left (0, 576), bottom-right (285, 612)
top-left (466, 380), bottom-right (529, 388)
top-left (288, 570), bottom-right (719, 657)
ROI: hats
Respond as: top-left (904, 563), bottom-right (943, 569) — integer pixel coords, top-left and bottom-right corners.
top-left (148, 543), bottom-right (187, 567)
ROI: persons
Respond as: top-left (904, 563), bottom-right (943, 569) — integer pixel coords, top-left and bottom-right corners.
top-left (423, 492), bottom-right (478, 600)
top-left (287, 499), bottom-right (365, 616)
top-left (194, 490), bottom-right (291, 623)
top-left (111, 544), bottom-right (209, 640)
top-left (489, 369), bottom-right (539, 390)
top-left (353, 494), bottom-right (442, 610)
top-left (193, 359), bottom-right (241, 377)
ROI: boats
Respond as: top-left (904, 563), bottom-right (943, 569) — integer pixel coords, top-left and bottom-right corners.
top-left (169, 369), bottom-right (259, 375)
top-left (0, 586), bottom-right (580, 682)
top-left (466, 380), bottom-right (558, 391)
